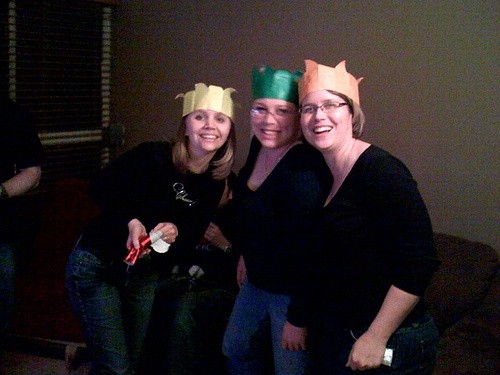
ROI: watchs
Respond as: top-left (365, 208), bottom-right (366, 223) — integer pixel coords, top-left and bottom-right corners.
top-left (224, 246), bottom-right (233, 253)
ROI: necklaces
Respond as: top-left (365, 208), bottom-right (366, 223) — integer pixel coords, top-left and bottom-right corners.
top-left (262, 148), bottom-right (290, 177)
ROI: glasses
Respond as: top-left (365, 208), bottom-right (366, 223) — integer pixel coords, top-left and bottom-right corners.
top-left (247, 104), bottom-right (298, 117)
top-left (298, 100), bottom-right (350, 114)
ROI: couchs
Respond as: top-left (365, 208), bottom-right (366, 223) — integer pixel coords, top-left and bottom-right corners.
top-left (5, 178), bottom-right (500, 375)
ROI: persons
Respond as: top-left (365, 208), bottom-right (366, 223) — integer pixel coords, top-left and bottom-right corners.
top-left (222, 66), bottom-right (329, 375)
top-left (65, 82), bottom-right (237, 375)
top-left (299, 57), bottom-right (439, 375)
top-left (0, 93), bottom-right (59, 333)
top-left (144, 169), bottom-right (238, 375)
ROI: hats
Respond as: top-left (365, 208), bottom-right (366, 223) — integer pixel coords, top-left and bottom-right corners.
top-left (175, 83), bottom-right (244, 123)
top-left (251, 63), bottom-right (304, 105)
top-left (294, 59), bottom-right (363, 105)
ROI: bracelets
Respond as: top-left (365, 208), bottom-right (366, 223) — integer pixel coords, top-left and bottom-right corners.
top-left (1, 184), bottom-right (9, 198)
